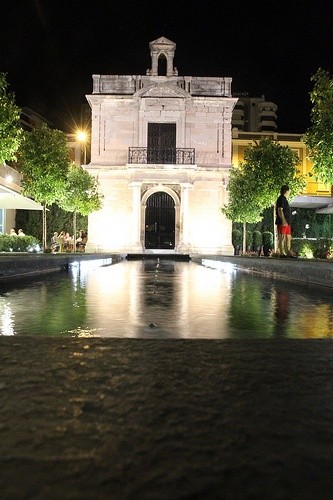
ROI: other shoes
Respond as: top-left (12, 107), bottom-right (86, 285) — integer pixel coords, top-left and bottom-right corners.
top-left (286, 254), bottom-right (297, 258)
top-left (279, 254), bottom-right (287, 258)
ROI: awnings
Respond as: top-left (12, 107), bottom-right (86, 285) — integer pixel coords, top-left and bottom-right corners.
top-left (0, 184), bottom-right (50, 210)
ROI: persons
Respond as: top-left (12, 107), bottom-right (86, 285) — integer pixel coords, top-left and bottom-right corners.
top-left (9, 229), bottom-right (26, 236)
top-left (52, 229), bottom-right (86, 252)
top-left (276, 185), bottom-right (298, 258)
